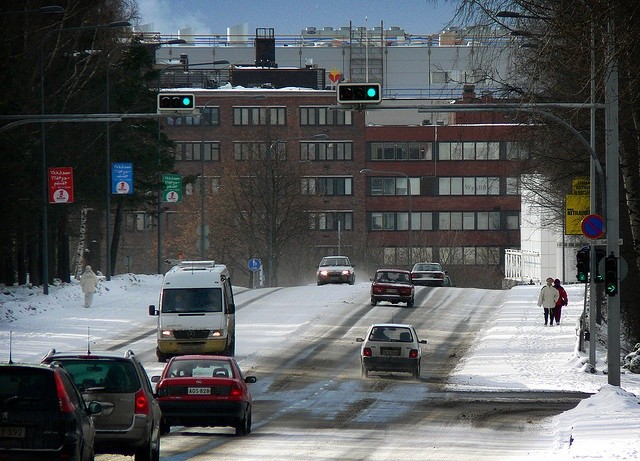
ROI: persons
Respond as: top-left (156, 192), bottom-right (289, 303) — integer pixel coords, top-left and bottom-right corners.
top-left (537, 277), bottom-right (560, 327)
top-left (80, 266), bottom-right (98, 308)
top-left (396, 274), bottom-right (409, 281)
top-left (379, 272), bottom-right (391, 281)
top-left (167, 294), bottom-right (190, 311)
top-left (183, 367), bottom-right (194, 377)
top-left (372, 327), bottom-right (389, 339)
top-left (552, 279), bottom-right (568, 325)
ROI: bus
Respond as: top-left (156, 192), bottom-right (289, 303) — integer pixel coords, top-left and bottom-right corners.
top-left (148, 260), bottom-right (236, 362)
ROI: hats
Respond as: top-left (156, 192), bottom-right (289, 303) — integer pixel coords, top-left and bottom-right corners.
top-left (546, 277), bottom-right (554, 282)
top-left (554, 278), bottom-right (560, 284)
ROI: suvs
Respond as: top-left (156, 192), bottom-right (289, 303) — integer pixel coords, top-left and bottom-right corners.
top-left (0, 329), bottom-right (103, 461)
top-left (38, 325), bottom-right (162, 461)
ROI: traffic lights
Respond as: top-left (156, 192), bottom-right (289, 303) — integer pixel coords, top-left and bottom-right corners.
top-left (606, 257), bottom-right (617, 297)
top-left (337, 82), bottom-right (382, 104)
top-left (596, 250), bottom-right (605, 282)
top-left (575, 251), bottom-right (587, 281)
top-left (157, 94), bottom-right (196, 110)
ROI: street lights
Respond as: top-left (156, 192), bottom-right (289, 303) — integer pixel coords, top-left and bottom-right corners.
top-left (157, 58), bottom-right (231, 273)
top-left (360, 168), bottom-right (412, 271)
top-left (105, 38), bottom-right (188, 280)
top-left (267, 133), bottom-right (328, 287)
top-left (200, 94), bottom-right (267, 259)
top-left (42, 20), bottom-right (132, 295)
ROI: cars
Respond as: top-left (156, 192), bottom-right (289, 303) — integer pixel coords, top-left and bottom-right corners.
top-left (315, 254), bottom-right (356, 285)
top-left (355, 323), bottom-right (427, 380)
top-left (369, 268), bottom-right (415, 309)
top-left (151, 354), bottom-right (257, 436)
top-left (411, 262), bottom-right (448, 286)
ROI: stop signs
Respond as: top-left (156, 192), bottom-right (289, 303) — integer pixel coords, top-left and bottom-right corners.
top-left (581, 214), bottom-right (606, 240)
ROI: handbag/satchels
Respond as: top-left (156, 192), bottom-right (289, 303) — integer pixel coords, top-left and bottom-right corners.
top-left (561, 298), bottom-right (565, 305)
